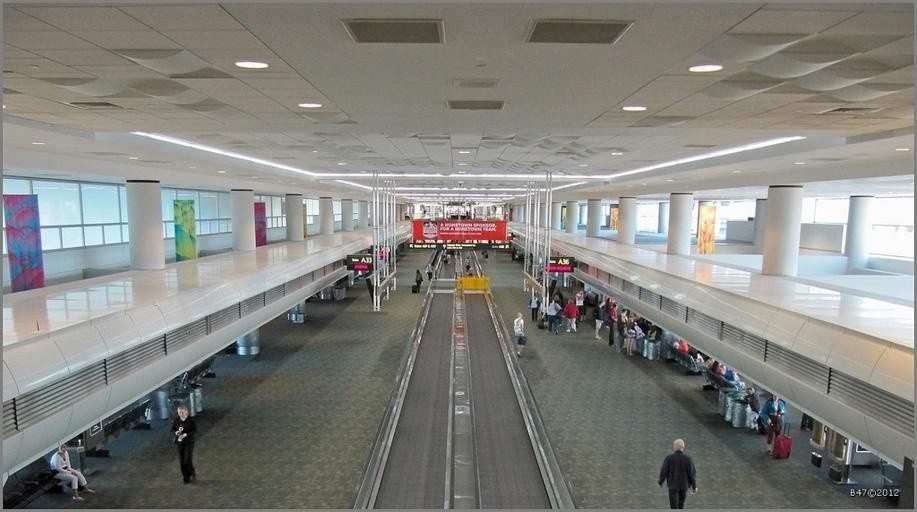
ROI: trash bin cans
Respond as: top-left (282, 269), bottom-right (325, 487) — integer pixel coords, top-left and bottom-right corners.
top-left (724, 395), bottom-right (748, 429)
top-left (183, 383), bottom-right (202, 417)
top-left (641, 336), bottom-right (657, 361)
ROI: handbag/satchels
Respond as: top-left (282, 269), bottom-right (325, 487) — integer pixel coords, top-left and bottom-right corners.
top-left (769, 416), bottom-right (782, 430)
top-left (518, 337), bottom-right (526, 345)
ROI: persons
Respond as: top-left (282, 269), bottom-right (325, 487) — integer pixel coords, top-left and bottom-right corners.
top-left (528, 289), bottom-right (813, 456)
top-left (50, 444), bottom-right (96, 501)
top-left (657, 439), bottom-right (698, 509)
top-left (443, 249), bottom-right (492, 277)
top-left (170, 404), bottom-right (197, 485)
top-left (514, 313), bottom-right (525, 357)
top-left (416, 270), bottom-right (423, 293)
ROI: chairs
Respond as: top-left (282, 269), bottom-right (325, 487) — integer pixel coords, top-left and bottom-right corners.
top-left (172, 355), bottom-right (216, 389)
top-left (1, 455), bottom-right (66, 509)
top-left (664, 343), bottom-right (743, 397)
top-left (100, 397), bottom-right (151, 441)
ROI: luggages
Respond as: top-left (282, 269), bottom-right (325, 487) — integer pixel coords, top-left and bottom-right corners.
top-left (772, 423), bottom-right (793, 458)
top-left (412, 284), bottom-right (417, 293)
